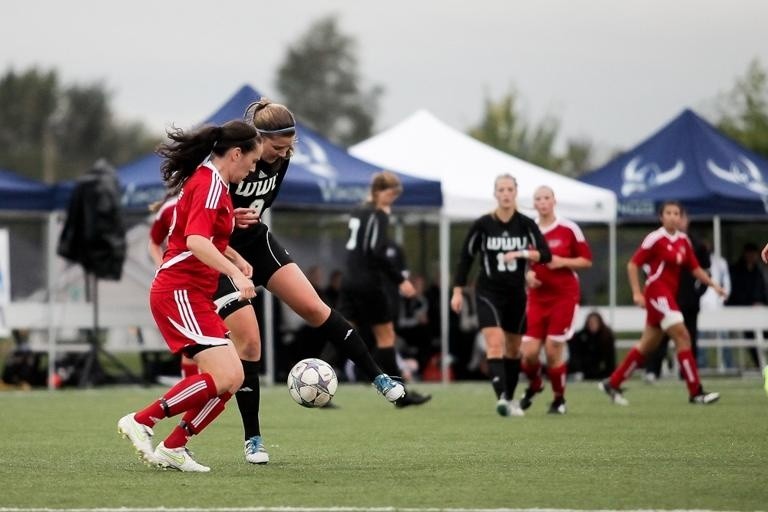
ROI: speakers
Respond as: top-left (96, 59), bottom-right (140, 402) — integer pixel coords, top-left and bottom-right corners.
top-left (57, 172), bottom-right (127, 280)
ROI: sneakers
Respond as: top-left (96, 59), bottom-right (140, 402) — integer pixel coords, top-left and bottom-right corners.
top-left (553, 394), bottom-right (566, 414)
top-left (600, 379), bottom-right (628, 404)
top-left (395, 391), bottom-right (431, 406)
top-left (520, 385), bottom-right (544, 410)
top-left (244, 436), bottom-right (270, 463)
top-left (118, 413), bottom-right (153, 461)
top-left (497, 398), bottom-right (513, 415)
top-left (372, 374), bottom-right (406, 402)
top-left (153, 442), bottom-right (210, 472)
top-left (691, 392), bottom-right (720, 404)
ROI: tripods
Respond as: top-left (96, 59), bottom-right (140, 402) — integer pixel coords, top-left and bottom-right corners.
top-left (64, 284), bottom-right (145, 392)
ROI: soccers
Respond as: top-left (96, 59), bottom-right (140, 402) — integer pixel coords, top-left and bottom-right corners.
top-left (288, 358), bottom-right (339, 409)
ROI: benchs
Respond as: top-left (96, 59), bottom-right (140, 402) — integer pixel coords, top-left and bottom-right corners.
top-left (1, 292), bottom-right (177, 391)
top-left (577, 306), bottom-right (768, 373)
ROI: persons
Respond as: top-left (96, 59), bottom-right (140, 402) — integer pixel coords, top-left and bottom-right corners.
top-left (642, 206), bottom-right (712, 382)
top-left (567, 312), bottom-right (617, 380)
top-left (450, 175), bottom-right (553, 418)
top-left (117, 121), bottom-right (257, 472)
top-left (181, 354), bottom-right (200, 380)
top-left (519, 186), bottom-right (594, 415)
top-left (321, 171), bottom-right (433, 408)
top-left (699, 241), bottom-right (768, 367)
top-left (597, 198), bottom-right (720, 408)
top-left (274, 268), bottom-right (362, 382)
top-left (394, 264), bottom-right (493, 381)
top-left (215, 97), bottom-right (405, 464)
